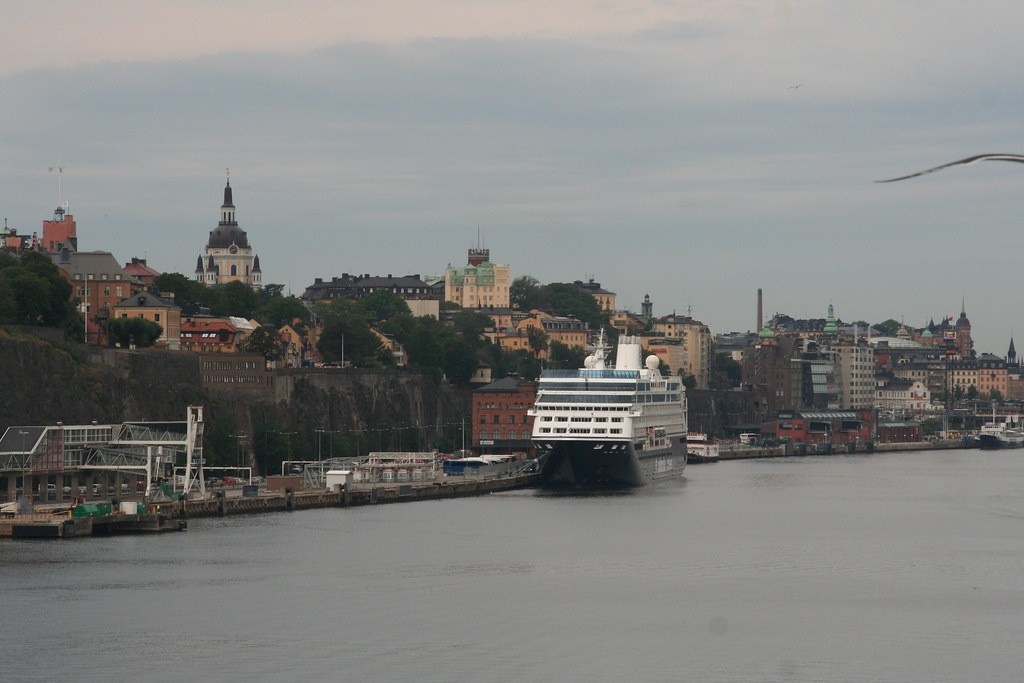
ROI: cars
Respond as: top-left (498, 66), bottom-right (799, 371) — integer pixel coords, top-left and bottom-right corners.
top-left (38, 481), bottom-right (144, 497)
top-left (193, 475), bottom-right (247, 488)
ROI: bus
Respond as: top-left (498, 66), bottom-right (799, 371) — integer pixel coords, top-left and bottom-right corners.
top-left (740, 434), bottom-right (757, 445)
top-left (443, 454), bottom-right (517, 476)
top-left (174, 466), bottom-right (252, 488)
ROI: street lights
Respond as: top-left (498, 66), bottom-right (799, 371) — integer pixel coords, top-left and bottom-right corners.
top-left (227, 434), bottom-right (247, 478)
top-left (371, 428), bottom-right (389, 452)
top-left (348, 429), bottom-right (367, 456)
top-left (447, 422), bottom-right (462, 453)
top-left (322, 429), bottom-right (341, 458)
top-left (392, 427), bottom-right (409, 452)
top-left (411, 425), bottom-right (427, 452)
top-left (18, 431), bottom-right (29, 501)
top-left (314, 429), bottom-right (325, 484)
top-left (279, 432), bottom-right (297, 476)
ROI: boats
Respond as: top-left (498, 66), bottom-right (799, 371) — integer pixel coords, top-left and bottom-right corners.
top-left (686, 431), bottom-right (719, 465)
top-left (978, 423), bottom-right (1024, 450)
top-left (525, 325), bottom-right (689, 493)
